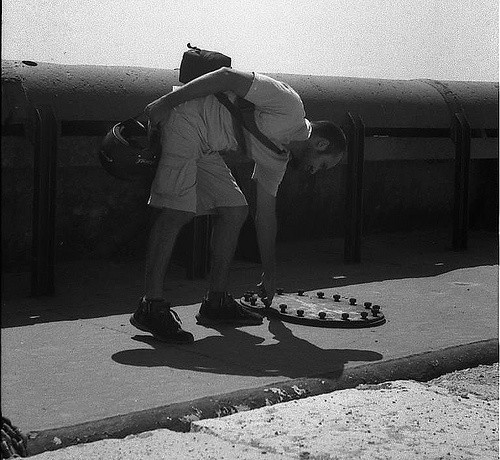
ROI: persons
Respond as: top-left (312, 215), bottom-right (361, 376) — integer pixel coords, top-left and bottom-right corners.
top-left (129, 66), bottom-right (347, 346)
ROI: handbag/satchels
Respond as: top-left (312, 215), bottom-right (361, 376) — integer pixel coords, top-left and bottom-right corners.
top-left (178, 43), bottom-right (232, 85)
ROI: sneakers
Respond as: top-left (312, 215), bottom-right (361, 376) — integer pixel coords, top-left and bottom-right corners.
top-left (196, 298), bottom-right (264, 327)
top-left (130, 298), bottom-right (195, 344)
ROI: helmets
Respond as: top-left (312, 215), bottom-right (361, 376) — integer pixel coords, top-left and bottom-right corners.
top-left (97, 121), bottom-right (160, 185)
